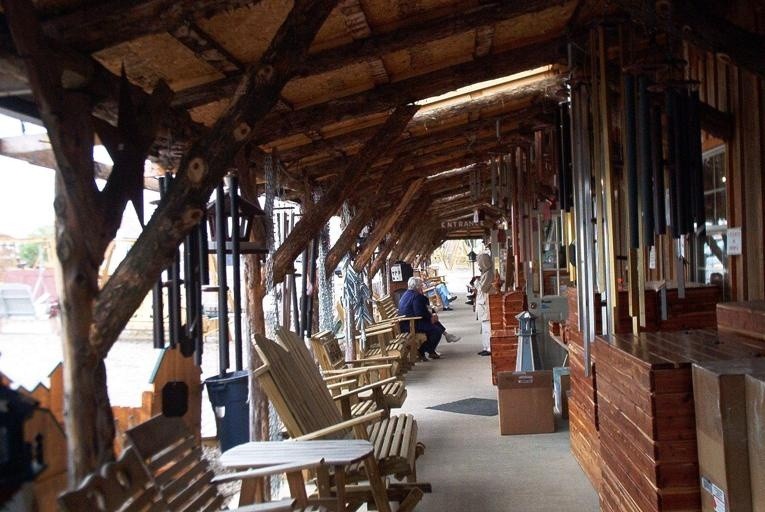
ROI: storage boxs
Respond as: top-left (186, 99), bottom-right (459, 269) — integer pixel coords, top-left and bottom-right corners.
top-left (691, 356), bottom-right (765, 512)
top-left (497, 366), bottom-right (570, 434)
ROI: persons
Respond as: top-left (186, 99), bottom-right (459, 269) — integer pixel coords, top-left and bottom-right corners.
top-left (397, 269), bottom-right (461, 361)
top-left (465, 275), bottom-right (483, 305)
top-left (472, 253), bottom-right (496, 357)
top-left (707, 271), bottom-right (723, 285)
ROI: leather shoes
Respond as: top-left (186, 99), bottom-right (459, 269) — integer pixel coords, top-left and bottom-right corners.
top-left (449, 296), bottom-right (457, 302)
top-left (443, 307), bottom-right (453, 310)
top-left (478, 351), bottom-right (491, 356)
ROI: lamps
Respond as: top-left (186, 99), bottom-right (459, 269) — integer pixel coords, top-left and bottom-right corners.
top-left (205, 191), bottom-right (269, 255)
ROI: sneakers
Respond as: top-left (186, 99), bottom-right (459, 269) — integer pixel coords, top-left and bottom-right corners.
top-left (429, 351), bottom-right (440, 358)
top-left (446, 334), bottom-right (461, 342)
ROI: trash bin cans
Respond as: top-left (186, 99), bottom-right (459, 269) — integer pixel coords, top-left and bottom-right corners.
top-left (204, 371), bottom-right (250, 450)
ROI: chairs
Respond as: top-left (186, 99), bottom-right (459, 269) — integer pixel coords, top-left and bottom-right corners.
top-left (54, 269), bottom-right (446, 512)
